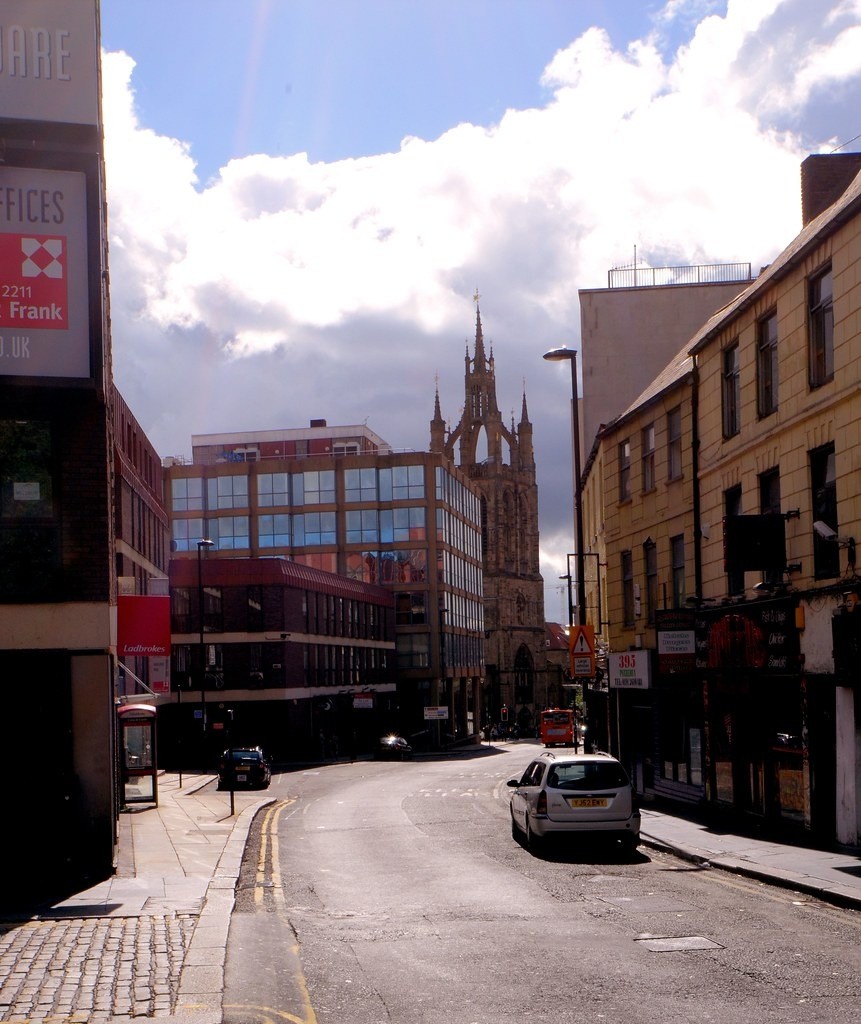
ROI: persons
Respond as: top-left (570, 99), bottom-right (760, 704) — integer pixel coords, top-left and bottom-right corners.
top-left (491, 723), bottom-right (519, 741)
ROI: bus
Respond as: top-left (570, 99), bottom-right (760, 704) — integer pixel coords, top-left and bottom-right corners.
top-left (539, 707), bottom-right (585, 747)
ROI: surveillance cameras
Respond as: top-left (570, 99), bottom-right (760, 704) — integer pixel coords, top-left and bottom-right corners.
top-left (812, 521), bottom-right (838, 542)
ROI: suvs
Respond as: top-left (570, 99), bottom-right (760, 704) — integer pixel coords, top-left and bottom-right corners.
top-left (217, 746), bottom-right (272, 791)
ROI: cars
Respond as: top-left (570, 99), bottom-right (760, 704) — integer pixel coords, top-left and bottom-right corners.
top-left (375, 735), bottom-right (413, 761)
top-left (506, 751), bottom-right (642, 852)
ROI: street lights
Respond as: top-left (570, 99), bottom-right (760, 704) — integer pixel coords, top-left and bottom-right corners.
top-left (557, 574), bottom-right (577, 755)
top-left (542, 344), bottom-right (594, 777)
top-left (196, 538), bottom-right (217, 774)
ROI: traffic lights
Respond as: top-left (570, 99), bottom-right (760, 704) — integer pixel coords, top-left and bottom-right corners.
top-left (501, 708), bottom-right (509, 722)
